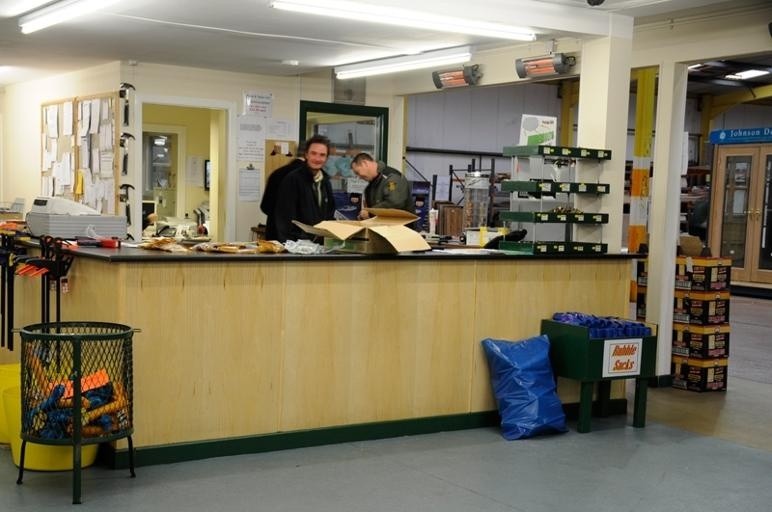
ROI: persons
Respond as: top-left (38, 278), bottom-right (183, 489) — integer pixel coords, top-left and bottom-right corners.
top-left (350, 153), bottom-right (417, 230)
top-left (261, 142), bottom-right (309, 243)
top-left (276, 135), bottom-right (337, 245)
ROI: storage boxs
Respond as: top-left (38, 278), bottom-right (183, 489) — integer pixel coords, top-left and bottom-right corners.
top-left (637, 258), bottom-right (732, 392)
top-left (292, 207), bottom-right (433, 256)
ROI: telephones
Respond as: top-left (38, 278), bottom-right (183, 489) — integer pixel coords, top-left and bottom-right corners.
top-left (156, 225), bottom-right (177, 237)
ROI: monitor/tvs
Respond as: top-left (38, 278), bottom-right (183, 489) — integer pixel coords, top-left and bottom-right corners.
top-left (205, 160), bottom-right (210, 191)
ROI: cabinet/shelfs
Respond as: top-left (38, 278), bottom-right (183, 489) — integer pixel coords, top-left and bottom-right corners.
top-left (708, 143), bottom-right (772, 299)
top-left (449, 159), bottom-right (510, 225)
top-left (499, 143), bottom-right (611, 254)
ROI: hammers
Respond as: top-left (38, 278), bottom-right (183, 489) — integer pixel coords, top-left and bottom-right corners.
top-left (120, 184), bottom-right (135, 225)
top-left (121, 133), bottom-right (136, 175)
top-left (121, 83), bottom-right (135, 126)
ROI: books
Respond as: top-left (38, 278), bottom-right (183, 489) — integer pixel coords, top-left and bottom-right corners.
top-left (46, 99), bottom-right (102, 199)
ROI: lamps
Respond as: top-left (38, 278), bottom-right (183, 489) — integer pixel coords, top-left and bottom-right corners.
top-left (330, 42), bottom-right (478, 80)
top-left (267, 1), bottom-right (537, 43)
top-left (18, 0), bottom-right (118, 36)
